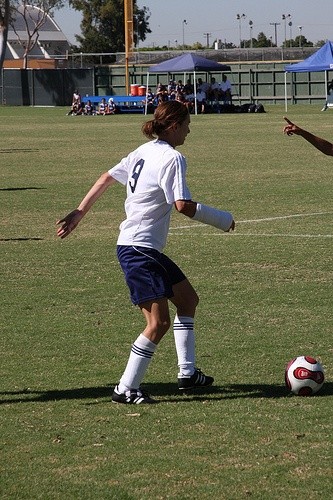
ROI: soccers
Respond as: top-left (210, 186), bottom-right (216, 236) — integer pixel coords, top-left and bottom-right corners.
top-left (285, 356), bottom-right (324, 396)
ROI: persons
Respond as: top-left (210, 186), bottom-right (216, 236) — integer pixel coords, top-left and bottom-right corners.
top-left (105, 98), bottom-right (116, 115)
top-left (98, 98), bottom-right (108, 115)
top-left (147, 75), bottom-right (231, 113)
top-left (283, 116), bottom-right (333, 156)
top-left (85, 100), bottom-right (96, 115)
top-left (56, 99), bottom-right (235, 404)
top-left (72, 90), bottom-right (82, 115)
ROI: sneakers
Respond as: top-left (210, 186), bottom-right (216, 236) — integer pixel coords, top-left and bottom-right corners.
top-left (177, 368), bottom-right (214, 390)
top-left (111, 385), bottom-right (156, 405)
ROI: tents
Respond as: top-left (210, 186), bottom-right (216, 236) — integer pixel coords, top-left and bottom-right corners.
top-left (145, 53), bottom-right (241, 115)
top-left (284, 41), bottom-right (333, 111)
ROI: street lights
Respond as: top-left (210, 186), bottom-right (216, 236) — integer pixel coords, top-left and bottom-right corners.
top-left (182, 20), bottom-right (187, 46)
top-left (282, 14), bottom-right (292, 47)
top-left (236, 13), bottom-right (245, 48)
